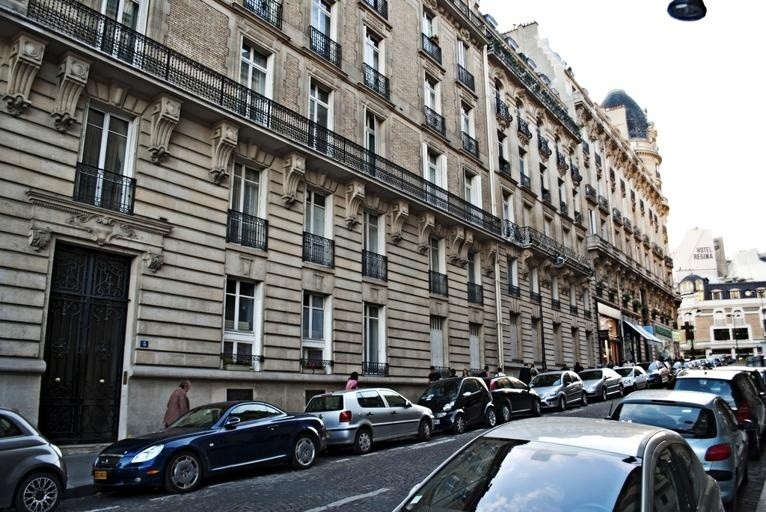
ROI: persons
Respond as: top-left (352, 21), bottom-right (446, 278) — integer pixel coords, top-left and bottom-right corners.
top-left (574, 362), bottom-right (583, 372)
top-left (427, 366), bottom-right (441, 380)
top-left (653, 356), bottom-right (685, 388)
top-left (561, 364), bottom-right (570, 370)
top-left (449, 368), bottom-right (457, 379)
top-left (461, 369), bottom-right (468, 380)
top-left (344, 371), bottom-right (359, 391)
top-left (495, 368), bottom-right (505, 377)
top-left (607, 359), bottom-right (637, 368)
top-left (162, 378), bottom-right (191, 428)
top-left (520, 363), bottom-right (539, 384)
top-left (482, 365), bottom-right (489, 377)
top-left (705, 354), bottom-right (764, 369)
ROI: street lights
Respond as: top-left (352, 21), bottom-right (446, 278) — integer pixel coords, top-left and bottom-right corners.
top-left (724, 313), bottom-right (742, 364)
top-left (743, 287), bottom-right (765, 325)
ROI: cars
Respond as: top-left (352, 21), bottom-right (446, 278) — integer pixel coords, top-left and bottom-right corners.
top-left (577, 367), bottom-right (625, 402)
top-left (529, 370), bottom-right (589, 411)
top-left (602, 387), bottom-right (751, 506)
top-left (301, 385), bottom-right (435, 453)
top-left (417, 376), bottom-right (497, 435)
top-left (0, 406), bottom-right (70, 512)
top-left (635, 353), bottom-right (766, 389)
top-left (611, 365), bottom-right (650, 393)
top-left (386, 411), bottom-right (732, 512)
top-left (483, 375), bottom-right (541, 422)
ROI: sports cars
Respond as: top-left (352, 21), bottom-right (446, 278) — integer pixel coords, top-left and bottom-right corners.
top-left (87, 394), bottom-right (326, 500)
